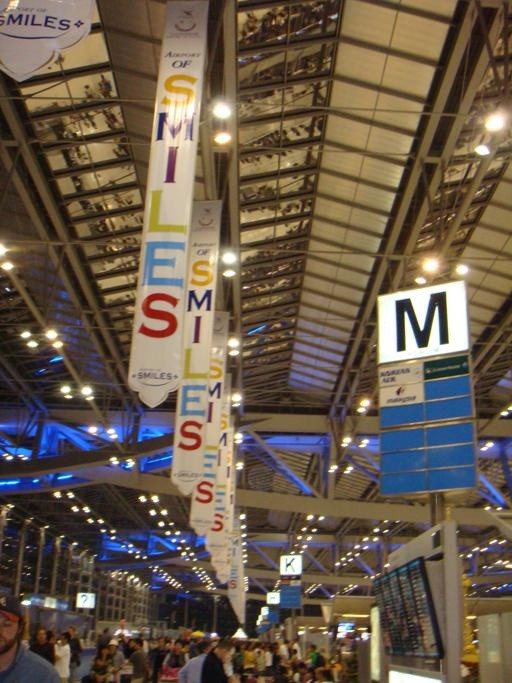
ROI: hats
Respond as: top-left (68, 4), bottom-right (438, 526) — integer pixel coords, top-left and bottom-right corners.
top-left (107, 639), bottom-right (118, 646)
top-left (0, 593), bottom-right (24, 622)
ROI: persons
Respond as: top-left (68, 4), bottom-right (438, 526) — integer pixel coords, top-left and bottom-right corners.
top-left (22, 627), bottom-right (361, 683)
top-left (0, 592), bottom-right (61, 682)
